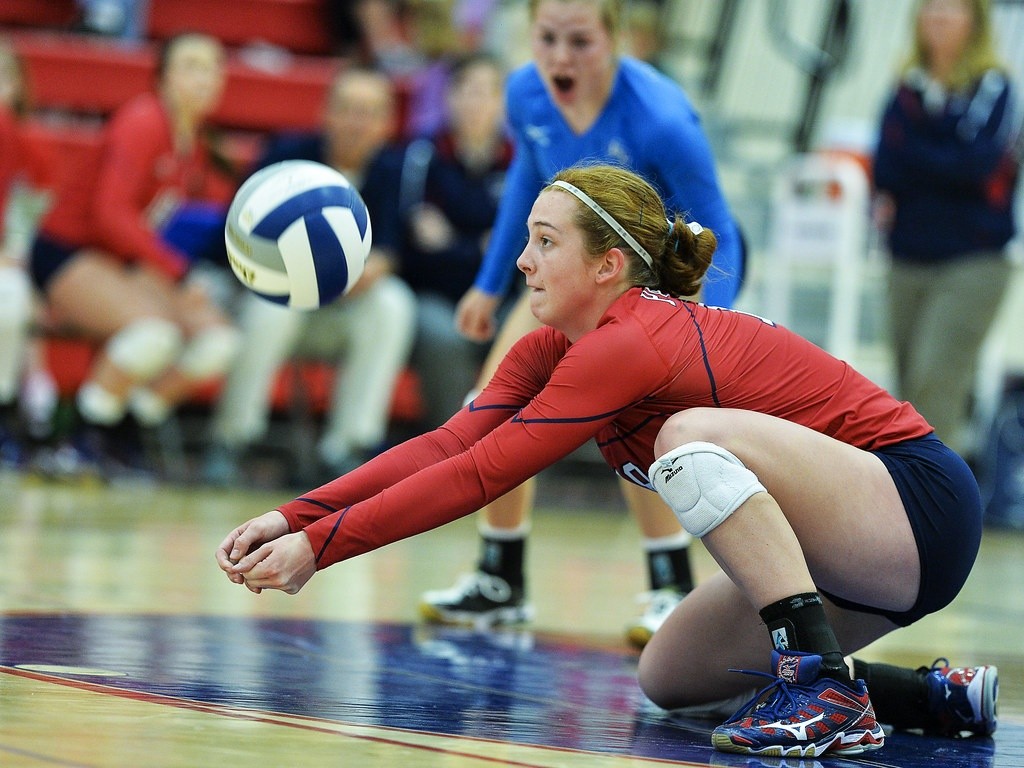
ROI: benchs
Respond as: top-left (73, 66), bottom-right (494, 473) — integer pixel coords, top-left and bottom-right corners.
top-left (0, 0), bottom-right (516, 444)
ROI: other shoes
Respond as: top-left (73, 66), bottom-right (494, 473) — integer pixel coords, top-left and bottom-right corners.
top-left (0, 402), bottom-right (69, 490)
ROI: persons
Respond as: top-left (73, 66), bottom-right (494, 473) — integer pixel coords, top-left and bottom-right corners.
top-left (0, 0), bottom-right (1023, 648)
top-left (214, 171), bottom-right (1002, 757)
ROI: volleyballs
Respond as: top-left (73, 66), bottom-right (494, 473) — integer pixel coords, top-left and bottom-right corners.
top-left (223, 158), bottom-right (374, 314)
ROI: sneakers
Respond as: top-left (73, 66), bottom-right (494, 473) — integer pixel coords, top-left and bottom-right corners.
top-left (61, 396), bottom-right (154, 488)
top-left (932, 662), bottom-right (998, 737)
top-left (627, 533), bottom-right (695, 644)
top-left (420, 526), bottom-right (532, 626)
top-left (710, 649), bottom-right (886, 756)
